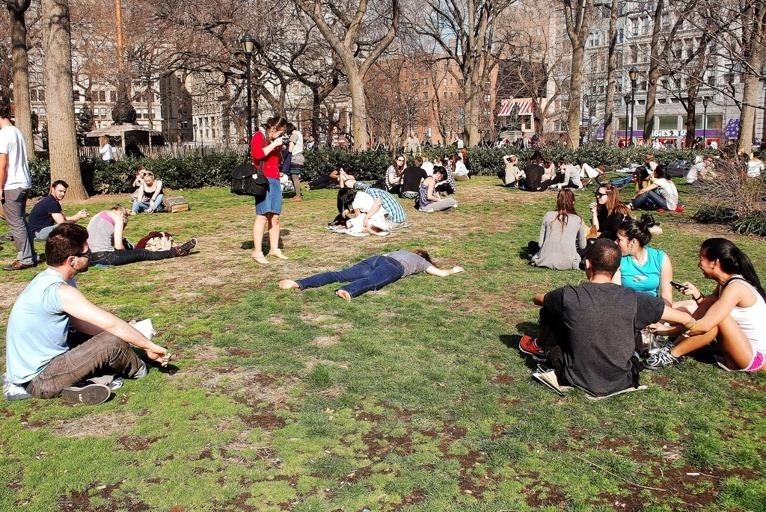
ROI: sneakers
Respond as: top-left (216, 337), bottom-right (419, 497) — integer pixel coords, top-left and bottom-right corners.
top-left (60, 382), bottom-right (111, 406)
top-left (518, 333), bottom-right (547, 363)
top-left (174, 237), bottom-right (198, 257)
top-left (641, 340), bottom-right (684, 371)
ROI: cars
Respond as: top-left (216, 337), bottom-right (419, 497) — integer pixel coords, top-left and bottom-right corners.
top-left (493, 131), bottom-right (763, 154)
top-left (306, 140), bottom-right (335, 151)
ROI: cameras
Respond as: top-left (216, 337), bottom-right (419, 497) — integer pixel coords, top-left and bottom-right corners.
top-left (282, 138), bottom-right (290, 146)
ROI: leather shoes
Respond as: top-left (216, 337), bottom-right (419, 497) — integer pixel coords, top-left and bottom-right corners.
top-left (1, 260), bottom-right (35, 272)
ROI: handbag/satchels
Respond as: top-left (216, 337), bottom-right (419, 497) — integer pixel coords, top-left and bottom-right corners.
top-left (230, 161), bottom-right (269, 195)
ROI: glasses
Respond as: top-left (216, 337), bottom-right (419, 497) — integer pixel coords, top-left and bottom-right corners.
top-left (596, 190), bottom-right (608, 198)
top-left (142, 172), bottom-right (153, 176)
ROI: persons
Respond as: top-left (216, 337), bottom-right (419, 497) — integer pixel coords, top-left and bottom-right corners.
top-left (99, 137), bottom-right (113, 161)
top-left (1, 103), bottom-right (36, 272)
top-left (27, 162), bottom-right (197, 266)
top-left (498, 150), bottom-right (765, 396)
top-left (420, 139), bottom-right (441, 148)
top-left (7, 224), bottom-right (172, 405)
top-left (479, 132), bottom-right (565, 150)
top-left (617, 134), bottom-right (714, 150)
top-left (752, 137), bottom-right (765, 150)
top-left (403, 132), bottom-right (419, 158)
top-left (304, 148), bottom-right (470, 234)
top-left (306, 135), bottom-right (319, 153)
top-left (278, 250), bottom-right (464, 303)
top-left (250, 115), bottom-right (304, 263)
top-left (454, 134), bottom-right (464, 148)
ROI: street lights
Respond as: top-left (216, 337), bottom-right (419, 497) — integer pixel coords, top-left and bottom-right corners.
top-left (346, 110), bottom-right (353, 149)
top-left (701, 98), bottom-right (709, 142)
top-left (623, 91), bottom-right (631, 147)
top-left (237, 29), bottom-right (256, 154)
top-left (628, 64), bottom-right (637, 143)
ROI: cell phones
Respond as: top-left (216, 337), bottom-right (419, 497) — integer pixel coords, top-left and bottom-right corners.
top-left (670, 281), bottom-right (688, 290)
